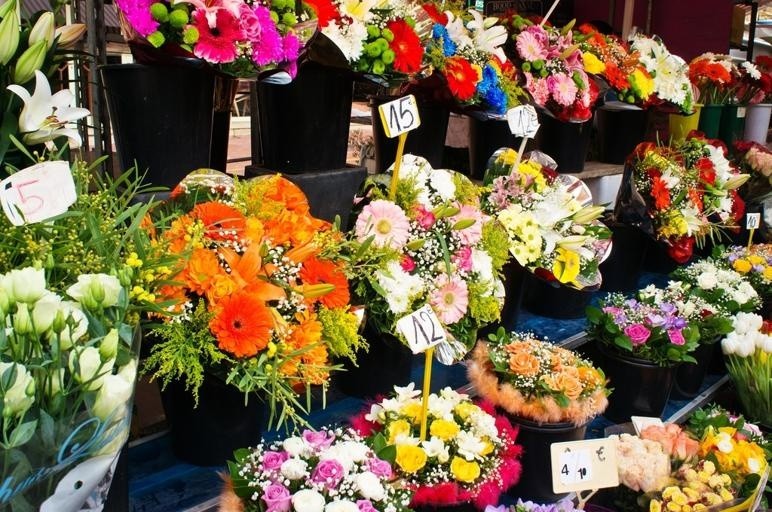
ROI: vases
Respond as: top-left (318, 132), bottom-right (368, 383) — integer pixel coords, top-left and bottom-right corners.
top-left (465, 108), bottom-right (533, 180)
top-left (525, 277), bottom-right (597, 322)
top-left (155, 374), bottom-right (272, 466)
top-left (330, 337), bottom-right (410, 406)
top-left (251, 62), bottom-right (353, 173)
top-left (724, 105), bottom-right (746, 147)
top-left (599, 103), bottom-right (653, 166)
top-left (479, 271), bottom-right (524, 337)
top-left (698, 105), bottom-right (725, 140)
top-left (666, 104), bottom-right (700, 143)
top-left (539, 104), bottom-right (589, 172)
top-left (98, 64), bottom-right (235, 190)
top-left (671, 346), bottom-right (706, 403)
top-left (747, 107), bottom-right (772, 147)
top-left (365, 93), bottom-right (451, 174)
top-left (599, 228), bottom-right (646, 293)
top-left (499, 413), bottom-right (589, 503)
top-left (596, 349), bottom-right (678, 424)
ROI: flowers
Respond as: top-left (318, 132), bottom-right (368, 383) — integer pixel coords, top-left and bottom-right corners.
top-left (639, 279), bottom-right (734, 345)
top-left (138, 170), bottom-right (402, 439)
top-left (713, 245), bottom-right (772, 291)
top-left (510, 25), bottom-right (598, 127)
top-left (351, 382), bottom-right (524, 511)
top-left (217, 423), bottom-right (414, 512)
top-left (424, 10), bottom-right (509, 122)
top-left (675, 259), bottom-right (764, 313)
top-left (0, 134), bottom-right (190, 512)
top-left (309, 5), bottom-right (428, 88)
top-left (686, 52), bottom-right (771, 107)
top-left (627, 29), bottom-right (698, 117)
top-left (720, 310), bottom-right (771, 423)
top-left (113, 0), bottom-right (302, 86)
top-left (0, 0), bottom-right (91, 166)
top-left (619, 139), bottom-right (706, 264)
top-left (348, 153), bottom-right (511, 364)
top-left (482, 146), bottom-right (614, 292)
top-left (606, 404), bottom-right (772, 511)
top-left (672, 135), bottom-right (752, 228)
top-left (462, 328), bottom-right (615, 427)
top-left (586, 28), bottom-right (656, 113)
